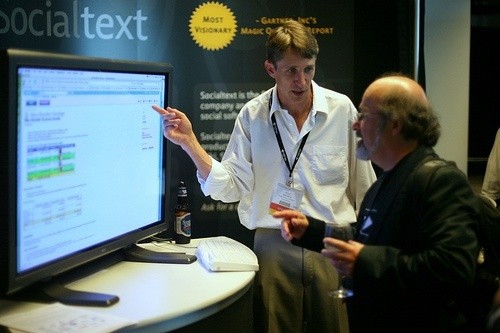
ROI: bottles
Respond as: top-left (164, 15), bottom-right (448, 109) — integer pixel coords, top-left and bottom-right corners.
top-left (173, 181), bottom-right (192, 244)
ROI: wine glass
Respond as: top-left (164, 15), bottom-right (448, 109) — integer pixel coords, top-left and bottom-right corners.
top-left (324, 220), bottom-right (354, 299)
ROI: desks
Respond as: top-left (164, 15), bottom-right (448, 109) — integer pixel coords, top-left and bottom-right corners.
top-left (0, 235), bottom-right (258, 333)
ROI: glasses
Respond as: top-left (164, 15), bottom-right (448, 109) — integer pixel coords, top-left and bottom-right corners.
top-left (356, 111), bottom-right (384, 122)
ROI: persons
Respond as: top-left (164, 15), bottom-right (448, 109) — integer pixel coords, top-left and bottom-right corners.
top-left (272, 71), bottom-right (481, 333)
top-left (481, 128), bottom-right (500, 208)
top-left (150, 19), bottom-right (378, 333)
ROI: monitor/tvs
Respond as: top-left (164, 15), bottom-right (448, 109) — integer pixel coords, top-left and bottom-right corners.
top-left (3, 48), bottom-right (196, 306)
